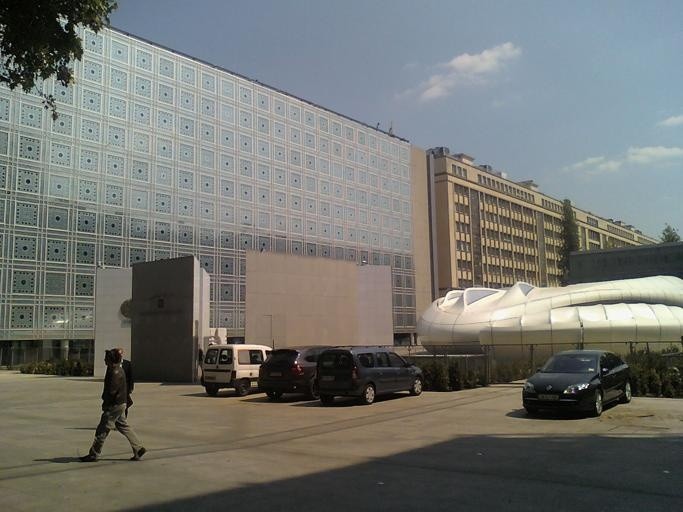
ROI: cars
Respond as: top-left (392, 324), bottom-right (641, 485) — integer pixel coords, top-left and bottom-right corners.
top-left (259, 346), bottom-right (339, 403)
top-left (520, 348), bottom-right (632, 419)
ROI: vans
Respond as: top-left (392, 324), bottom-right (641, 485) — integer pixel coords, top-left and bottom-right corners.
top-left (199, 343), bottom-right (276, 396)
top-left (314, 347), bottom-right (425, 404)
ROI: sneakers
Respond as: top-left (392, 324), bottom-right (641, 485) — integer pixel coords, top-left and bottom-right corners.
top-left (130, 448), bottom-right (148, 461)
top-left (79, 454), bottom-right (99, 462)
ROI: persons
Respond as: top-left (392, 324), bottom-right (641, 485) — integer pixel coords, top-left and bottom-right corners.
top-left (78, 348), bottom-right (148, 462)
